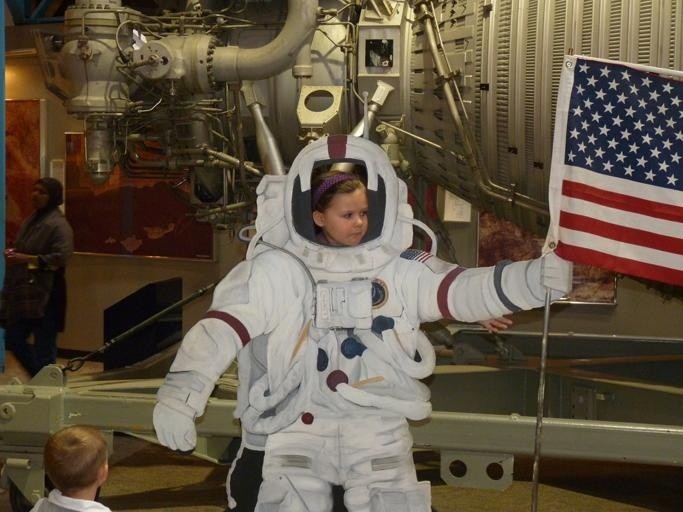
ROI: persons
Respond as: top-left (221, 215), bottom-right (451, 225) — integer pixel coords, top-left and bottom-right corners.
top-left (28, 427), bottom-right (115, 511)
top-left (3, 177), bottom-right (74, 374)
top-left (154, 170), bottom-right (574, 512)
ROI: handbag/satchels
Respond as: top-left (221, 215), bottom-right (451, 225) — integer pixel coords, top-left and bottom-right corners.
top-left (0, 261), bottom-right (54, 327)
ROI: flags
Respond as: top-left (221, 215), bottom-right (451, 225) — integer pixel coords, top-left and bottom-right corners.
top-left (545, 57), bottom-right (682, 290)
